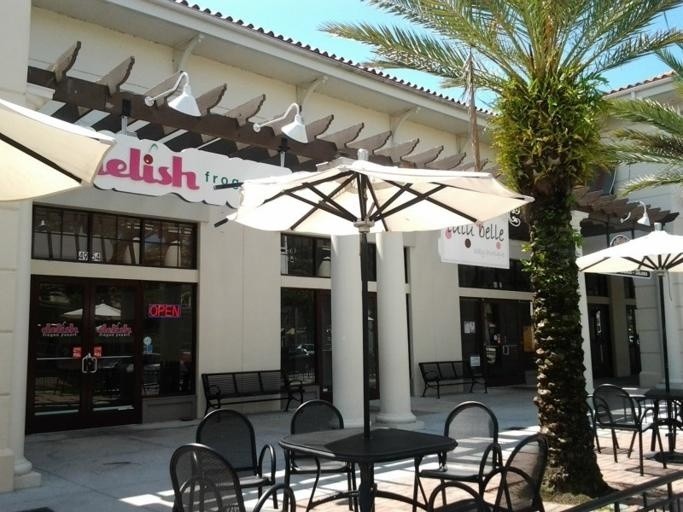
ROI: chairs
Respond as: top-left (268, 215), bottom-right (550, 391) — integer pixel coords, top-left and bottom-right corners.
top-left (282, 399), bottom-right (359, 512)
top-left (411, 399), bottom-right (511, 512)
top-left (586, 381), bottom-right (683, 476)
top-left (169, 443), bottom-right (297, 512)
top-left (430, 434), bottom-right (549, 511)
top-left (189, 407), bottom-right (278, 512)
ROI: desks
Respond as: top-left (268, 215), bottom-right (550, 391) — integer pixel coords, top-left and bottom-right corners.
top-left (279, 426), bottom-right (457, 512)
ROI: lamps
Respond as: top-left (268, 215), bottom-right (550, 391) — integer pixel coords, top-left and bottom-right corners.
top-left (618, 201), bottom-right (651, 228)
top-left (253, 103), bottom-right (308, 144)
top-left (143, 70), bottom-right (202, 118)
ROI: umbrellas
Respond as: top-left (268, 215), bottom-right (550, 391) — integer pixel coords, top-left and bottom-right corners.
top-left (208, 155), bottom-right (538, 438)
top-left (575, 230), bottom-right (683, 433)
top-left (0, 98), bottom-right (118, 204)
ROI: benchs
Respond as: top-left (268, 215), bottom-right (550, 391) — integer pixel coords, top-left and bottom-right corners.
top-left (419, 360), bottom-right (488, 397)
top-left (202, 370), bottom-right (305, 419)
